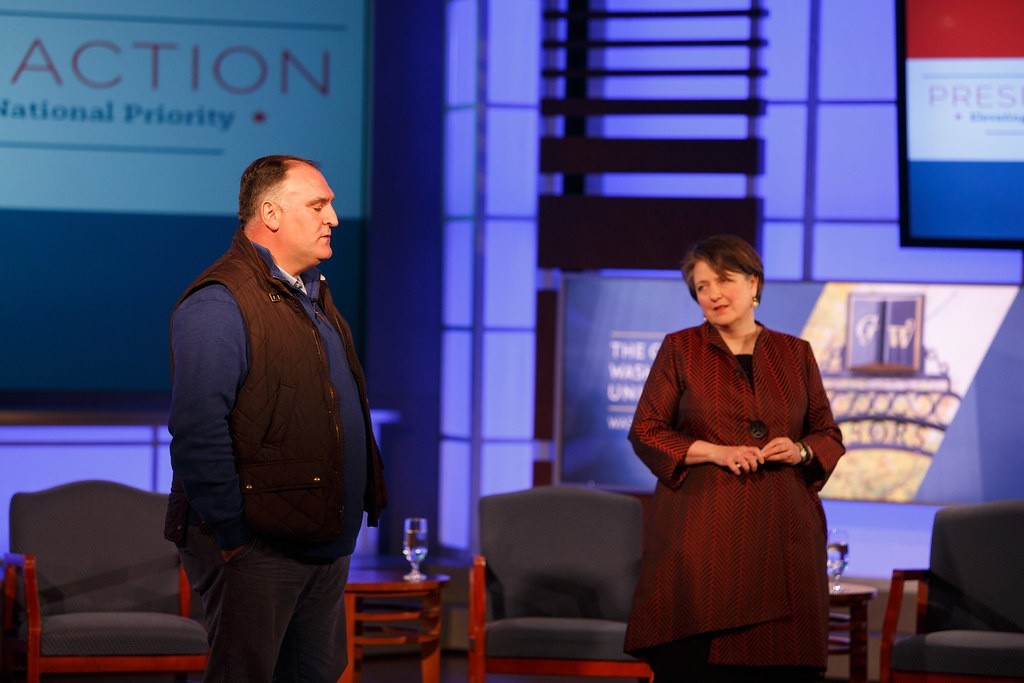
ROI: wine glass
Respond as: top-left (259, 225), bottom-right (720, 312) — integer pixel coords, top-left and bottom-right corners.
top-left (827, 528), bottom-right (850, 592)
top-left (403, 517), bottom-right (428, 581)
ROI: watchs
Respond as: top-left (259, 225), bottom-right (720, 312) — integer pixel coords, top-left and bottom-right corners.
top-left (795, 441), bottom-right (810, 465)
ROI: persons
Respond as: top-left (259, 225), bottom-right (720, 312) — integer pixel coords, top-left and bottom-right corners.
top-left (621, 233), bottom-right (846, 682)
top-left (165, 153), bottom-right (393, 683)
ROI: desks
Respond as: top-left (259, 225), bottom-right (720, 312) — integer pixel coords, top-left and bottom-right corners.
top-left (827, 584), bottom-right (880, 683)
top-left (337, 570), bottom-right (449, 683)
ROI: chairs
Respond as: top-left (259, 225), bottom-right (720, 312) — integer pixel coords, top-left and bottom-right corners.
top-left (880, 499), bottom-right (1024, 683)
top-left (4, 478), bottom-right (212, 683)
top-left (468, 487), bottom-right (654, 683)
